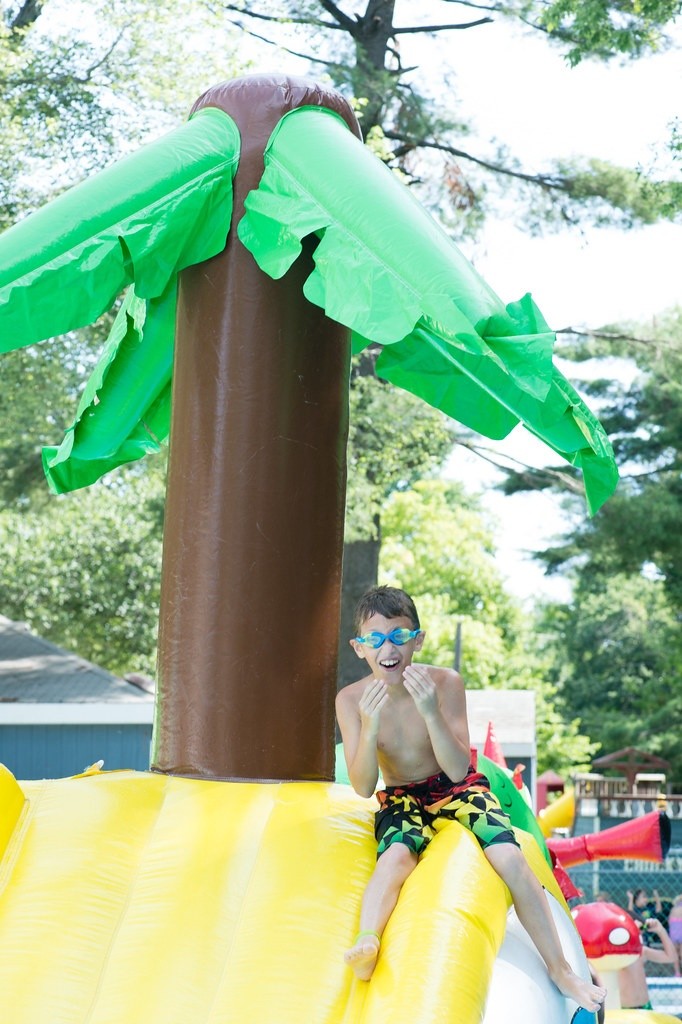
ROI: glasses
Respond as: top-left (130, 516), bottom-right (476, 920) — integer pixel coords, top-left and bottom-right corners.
top-left (355, 628), bottom-right (421, 648)
top-left (638, 923), bottom-right (650, 930)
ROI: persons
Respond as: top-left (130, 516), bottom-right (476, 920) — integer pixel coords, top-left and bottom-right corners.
top-left (656, 800), bottom-right (671, 868)
top-left (332, 584), bottom-right (606, 1012)
top-left (593, 888), bottom-right (671, 948)
top-left (609, 912), bottom-right (678, 1011)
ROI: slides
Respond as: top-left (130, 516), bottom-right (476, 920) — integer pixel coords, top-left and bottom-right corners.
top-left (0, 754), bottom-right (604, 1023)
